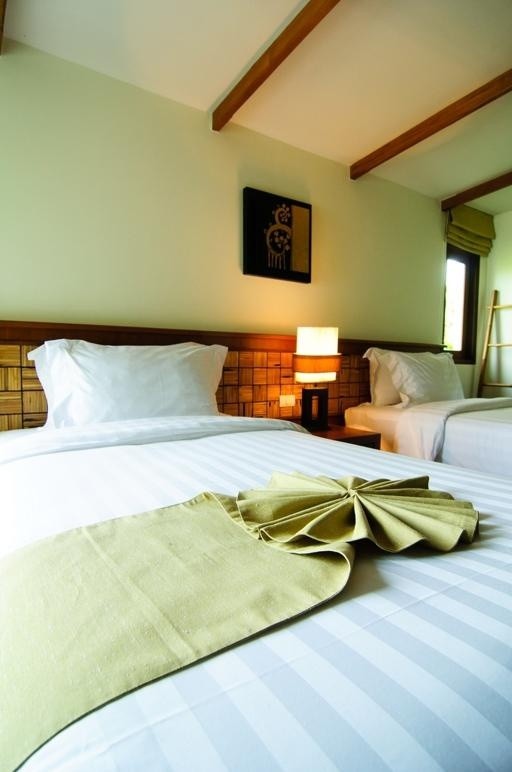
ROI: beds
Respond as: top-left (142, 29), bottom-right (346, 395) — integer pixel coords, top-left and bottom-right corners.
top-left (345, 387), bottom-right (511, 467)
top-left (0, 415), bottom-right (510, 769)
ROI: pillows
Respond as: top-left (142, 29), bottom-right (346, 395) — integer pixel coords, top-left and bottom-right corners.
top-left (51, 339), bottom-right (225, 428)
top-left (384, 351), bottom-right (468, 401)
top-left (360, 345), bottom-right (414, 412)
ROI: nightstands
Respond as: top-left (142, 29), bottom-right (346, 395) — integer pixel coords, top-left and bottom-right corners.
top-left (282, 412), bottom-right (384, 451)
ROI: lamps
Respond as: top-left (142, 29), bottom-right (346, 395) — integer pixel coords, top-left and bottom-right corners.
top-left (290, 319), bottom-right (342, 429)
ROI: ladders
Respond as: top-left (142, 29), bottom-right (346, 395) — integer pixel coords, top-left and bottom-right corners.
top-left (477, 290), bottom-right (512, 398)
